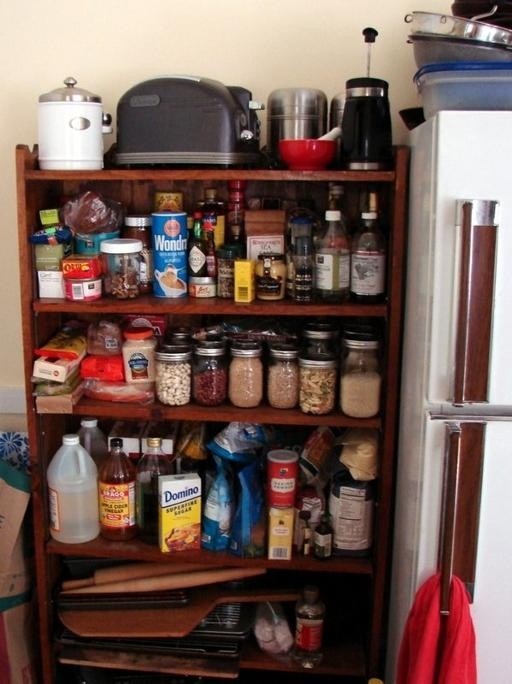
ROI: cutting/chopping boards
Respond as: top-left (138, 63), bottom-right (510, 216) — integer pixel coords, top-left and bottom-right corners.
top-left (60, 591), bottom-right (301, 638)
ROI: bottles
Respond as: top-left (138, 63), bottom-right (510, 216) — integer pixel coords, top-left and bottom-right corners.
top-left (290, 586), bottom-right (327, 670)
top-left (258, 180), bottom-right (386, 304)
top-left (186, 177), bottom-right (247, 297)
top-left (135, 437), bottom-right (175, 544)
top-left (95, 436), bottom-right (136, 540)
top-left (120, 322), bottom-right (383, 421)
top-left (313, 513), bottom-right (333, 561)
top-left (96, 237), bottom-right (145, 301)
top-left (296, 512), bottom-right (311, 556)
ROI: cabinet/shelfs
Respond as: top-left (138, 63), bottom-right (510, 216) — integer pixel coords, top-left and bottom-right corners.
top-left (15, 139), bottom-right (412, 683)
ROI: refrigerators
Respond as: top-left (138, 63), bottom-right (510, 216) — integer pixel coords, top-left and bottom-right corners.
top-left (384, 105), bottom-right (508, 682)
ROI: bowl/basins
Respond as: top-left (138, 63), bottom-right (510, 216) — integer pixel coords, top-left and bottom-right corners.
top-left (278, 139), bottom-right (339, 171)
top-left (402, 8), bottom-right (511, 66)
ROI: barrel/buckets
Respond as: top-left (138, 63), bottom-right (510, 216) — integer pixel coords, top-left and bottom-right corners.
top-left (45, 433), bottom-right (102, 547)
top-left (79, 419), bottom-right (105, 456)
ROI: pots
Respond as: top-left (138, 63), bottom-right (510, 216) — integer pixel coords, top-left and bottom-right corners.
top-left (37, 77), bottom-right (113, 168)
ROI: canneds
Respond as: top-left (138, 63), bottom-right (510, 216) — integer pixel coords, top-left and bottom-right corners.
top-left (123, 324), bottom-right (380, 419)
top-left (267, 448), bottom-right (299, 509)
top-left (256, 252), bottom-right (286, 301)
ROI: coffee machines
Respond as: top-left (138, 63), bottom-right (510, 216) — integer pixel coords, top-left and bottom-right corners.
top-left (341, 77), bottom-right (393, 172)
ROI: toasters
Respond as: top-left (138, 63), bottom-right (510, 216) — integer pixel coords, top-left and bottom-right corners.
top-left (114, 73), bottom-right (263, 167)
